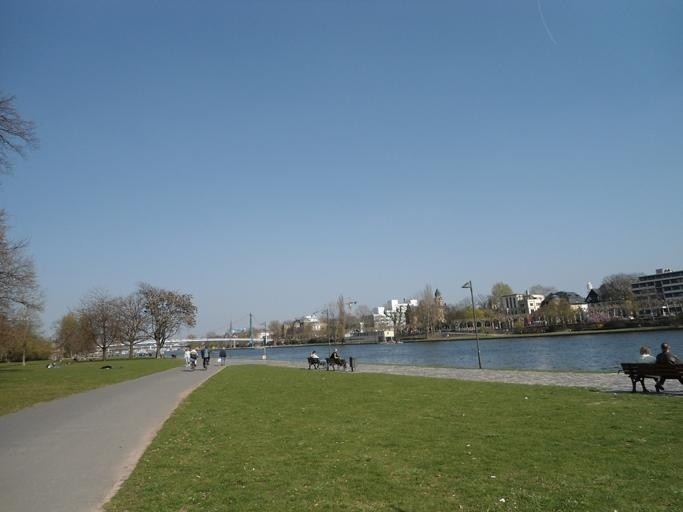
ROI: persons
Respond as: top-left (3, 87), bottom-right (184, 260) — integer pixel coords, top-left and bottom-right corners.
top-left (655, 342), bottom-right (683, 392)
top-left (330, 348), bottom-right (350, 371)
top-left (310, 351), bottom-right (327, 368)
top-left (638, 346), bottom-right (664, 392)
top-left (44, 347), bottom-right (227, 371)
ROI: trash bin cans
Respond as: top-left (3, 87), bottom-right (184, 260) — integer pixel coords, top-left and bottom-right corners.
top-left (350, 357), bottom-right (356, 372)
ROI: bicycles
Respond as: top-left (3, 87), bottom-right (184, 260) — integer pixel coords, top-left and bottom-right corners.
top-left (191, 359), bottom-right (208, 371)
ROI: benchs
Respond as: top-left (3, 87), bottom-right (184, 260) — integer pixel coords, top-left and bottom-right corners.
top-left (616, 361), bottom-right (683, 393)
top-left (308, 358), bottom-right (348, 372)
